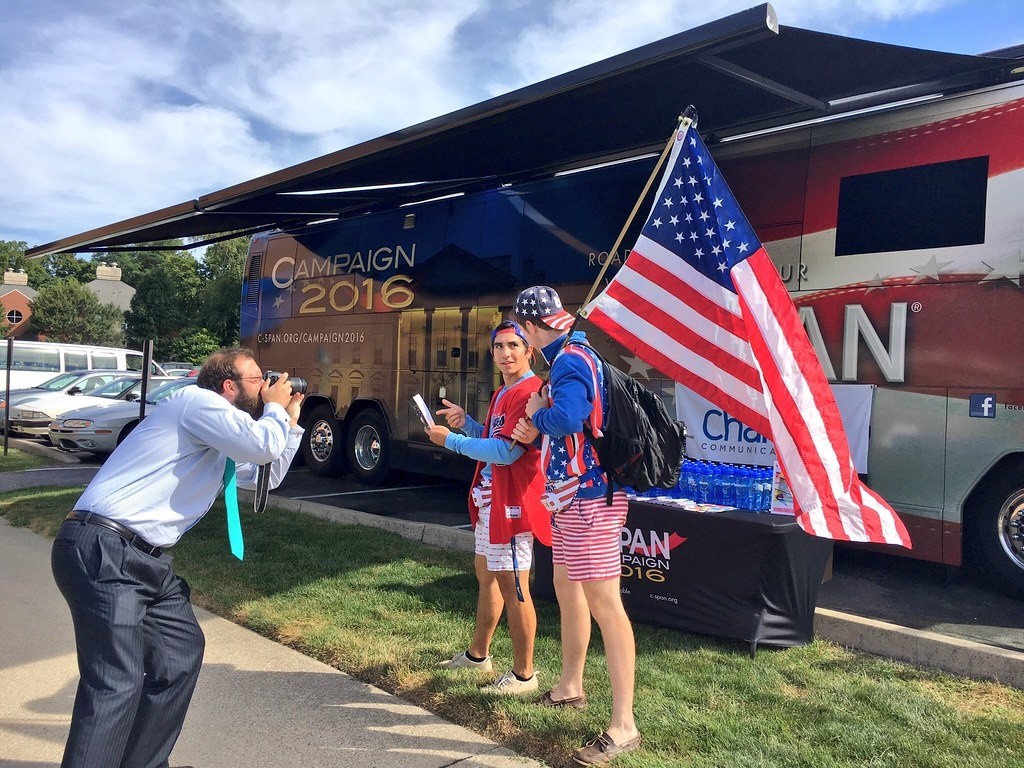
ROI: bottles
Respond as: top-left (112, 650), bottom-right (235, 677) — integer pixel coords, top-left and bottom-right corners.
top-left (618, 457), bottom-right (774, 511)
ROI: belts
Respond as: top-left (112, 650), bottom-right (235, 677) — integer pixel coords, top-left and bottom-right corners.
top-left (65, 511), bottom-right (162, 558)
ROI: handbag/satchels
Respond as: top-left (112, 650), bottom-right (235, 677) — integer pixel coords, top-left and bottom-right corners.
top-left (470, 485), bottom-right (492, 509)
top-left (539, 467), bottom-right (602, 512)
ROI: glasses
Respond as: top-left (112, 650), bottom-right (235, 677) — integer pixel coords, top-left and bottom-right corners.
top-left (236, 377), bottom-right (263, 384)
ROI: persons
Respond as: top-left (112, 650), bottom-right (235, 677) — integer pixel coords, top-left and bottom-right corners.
top-left (510, 287), bottom-right (643, 765)
top-left (50, 342), bottom-right (308, 768)
top-left (422, 317), bottom-right (553, 694)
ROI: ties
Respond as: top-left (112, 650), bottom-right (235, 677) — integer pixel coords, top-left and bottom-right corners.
top-left (224, 455), bottom-right (244, 561)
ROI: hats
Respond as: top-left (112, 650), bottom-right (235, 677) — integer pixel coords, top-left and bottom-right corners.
top-left (517, 286), bottom-right (575, 330)
top-left (490, 321), bottom-right (536, 367)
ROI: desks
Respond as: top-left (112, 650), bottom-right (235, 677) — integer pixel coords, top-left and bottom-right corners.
top-left (531, 495), bottom-right (831, 658)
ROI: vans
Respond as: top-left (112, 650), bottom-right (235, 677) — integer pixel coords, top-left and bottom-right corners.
top-left (0, 340), bottom-right (170, 399)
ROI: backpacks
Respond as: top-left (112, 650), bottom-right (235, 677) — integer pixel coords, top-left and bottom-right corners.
top-left (551, 340), bottom-right (688, 492)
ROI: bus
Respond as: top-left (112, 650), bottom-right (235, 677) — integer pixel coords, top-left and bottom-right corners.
top-left (236, 77), bottom-right (1024, 601)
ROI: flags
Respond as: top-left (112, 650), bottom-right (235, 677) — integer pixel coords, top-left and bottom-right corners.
top-left (582, 116), bottom-right (912, 550)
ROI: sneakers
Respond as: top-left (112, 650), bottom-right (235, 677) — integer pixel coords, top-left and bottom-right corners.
top-left (444, 651), bottom-right (494, 671)
top-left (479, 670), bottom-right (540, 694)
top-left (531, 688), bottom-right (587, 710)
top-left (571, 727), bottom-right (640, 766)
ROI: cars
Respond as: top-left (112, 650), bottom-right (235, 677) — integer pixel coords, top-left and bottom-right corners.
top-left (48, 376), bottom-right (199, 458)
top-left (158, 361), bottom-right (201, 378)
top-left (0, 370), bottom-right (145, 443)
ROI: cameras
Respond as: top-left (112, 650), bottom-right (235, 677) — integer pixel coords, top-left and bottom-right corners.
top-left (263, 370), bottom-right (308, 396)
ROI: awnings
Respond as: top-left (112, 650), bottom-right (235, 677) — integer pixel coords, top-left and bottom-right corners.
top-left (22, 2), bottom-right (1024, 258)
